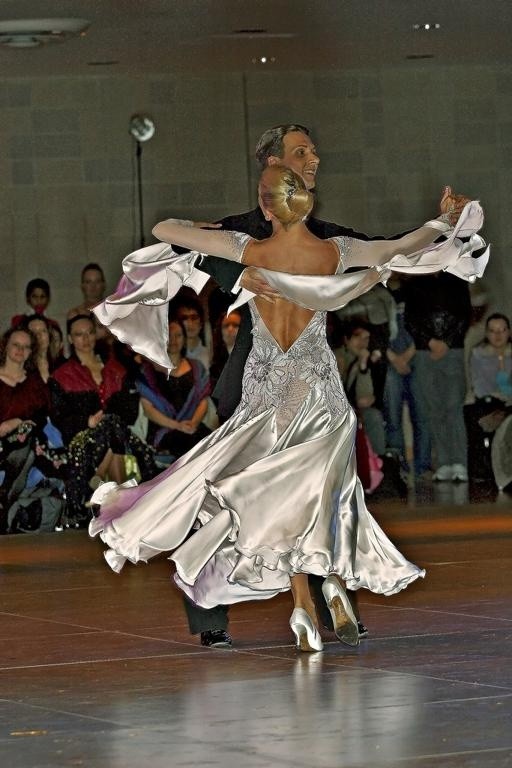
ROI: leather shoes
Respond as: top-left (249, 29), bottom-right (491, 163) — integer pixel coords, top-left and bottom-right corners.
top-left (201, 628), bottom-right (231, 648)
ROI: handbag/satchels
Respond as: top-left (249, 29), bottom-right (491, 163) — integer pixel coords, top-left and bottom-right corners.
top-left (382, 450), bottom-right (408, 506)
top-left (7, 480), bottom-right (67, 535)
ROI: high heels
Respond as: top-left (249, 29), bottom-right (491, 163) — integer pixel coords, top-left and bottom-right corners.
top-left (289, 607), bottom-right (325, 654)
top-left (321, 575), bottom-right (359, 647)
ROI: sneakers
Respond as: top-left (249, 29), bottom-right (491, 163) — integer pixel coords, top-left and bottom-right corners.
top-left (415, 463), bottom-right (469, 483)
top-left (321, 621), bottom-right (369, 639)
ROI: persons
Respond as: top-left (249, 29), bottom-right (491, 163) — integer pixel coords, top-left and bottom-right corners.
top-left (87, 160), bottom-right (494, 655)
top-left (324, 235), bottom-right (512, 494)
top-left (0, 262), bottom-right (241, 534)
top-left (147, 122), bottom-right (492, 650)
